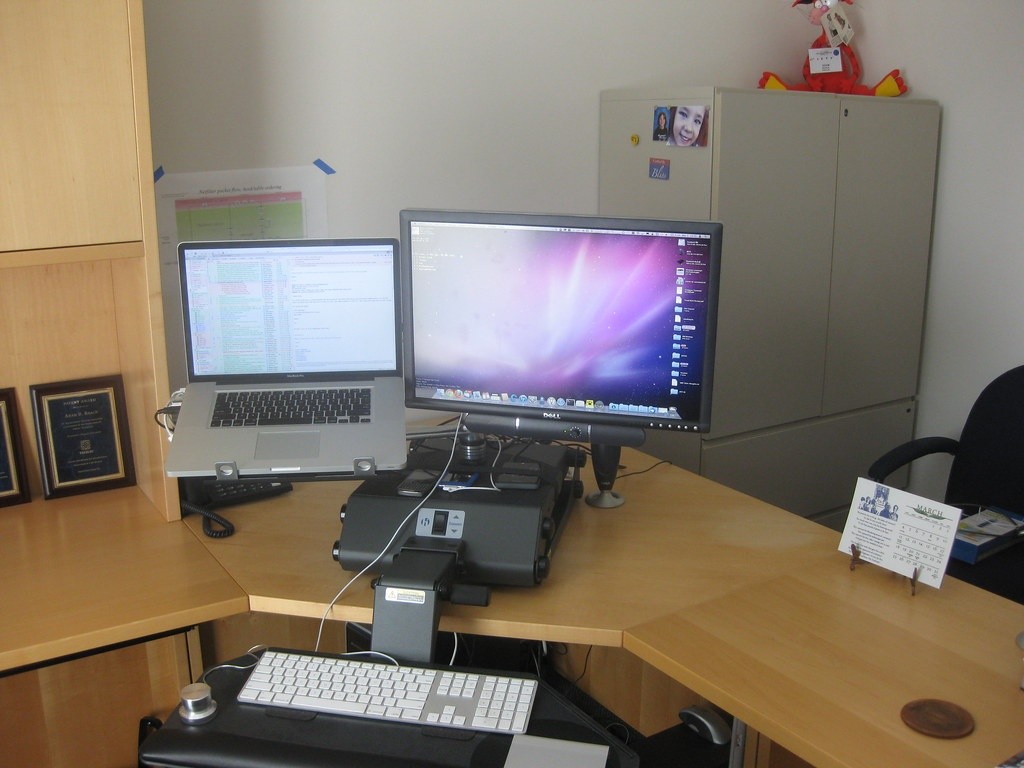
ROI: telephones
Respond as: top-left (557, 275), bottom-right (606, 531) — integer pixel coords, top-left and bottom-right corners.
top-left (177, 478), bottom-right (293, 517)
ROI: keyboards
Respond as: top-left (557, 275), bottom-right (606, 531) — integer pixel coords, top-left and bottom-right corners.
top-left (237, 650), bottom-right (537, 735)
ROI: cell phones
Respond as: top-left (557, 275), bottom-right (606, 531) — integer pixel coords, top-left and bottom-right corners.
top-left (397, 469), bottom-right (443, 497)
top-left (495, 474), bottom-right (541, 489)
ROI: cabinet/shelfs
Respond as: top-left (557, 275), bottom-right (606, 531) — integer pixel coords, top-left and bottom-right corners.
top-left (702, 399), bottom-right (916, 519)
top-left (0, 0), bottom-right (158, 268)
top-left (597, 85), bottom-right (942, 440)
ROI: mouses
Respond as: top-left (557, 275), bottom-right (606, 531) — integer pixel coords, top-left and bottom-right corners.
top-left (678, 705), bottom-right (732, 744)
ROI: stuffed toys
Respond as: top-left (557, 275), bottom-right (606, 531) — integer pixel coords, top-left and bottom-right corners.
top-left (757, 0), bottom-right (907, 97)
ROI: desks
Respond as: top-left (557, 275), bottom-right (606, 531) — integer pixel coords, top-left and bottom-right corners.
top-left (622, 553), bottom-right (1024, 768)
top-left (2, 481), bottom-right (249, 768)
top-left (180, 412), bottom-right (843, 739)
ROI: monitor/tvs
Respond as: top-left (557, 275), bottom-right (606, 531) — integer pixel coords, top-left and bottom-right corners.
top-left (396, 208), bottom-right (724, 448)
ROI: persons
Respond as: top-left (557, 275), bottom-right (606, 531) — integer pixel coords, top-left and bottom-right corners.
top-left (668, 106), bottom-right (708, 146)
top-left (653, 111), bottom-right (670, 141)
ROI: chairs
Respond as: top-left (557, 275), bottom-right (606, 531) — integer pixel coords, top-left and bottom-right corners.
top-left (867, 364), bottom-right (1024, 604)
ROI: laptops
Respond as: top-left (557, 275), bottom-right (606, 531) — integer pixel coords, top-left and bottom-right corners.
top-left (165, 238), bottom-right (408, 478)
